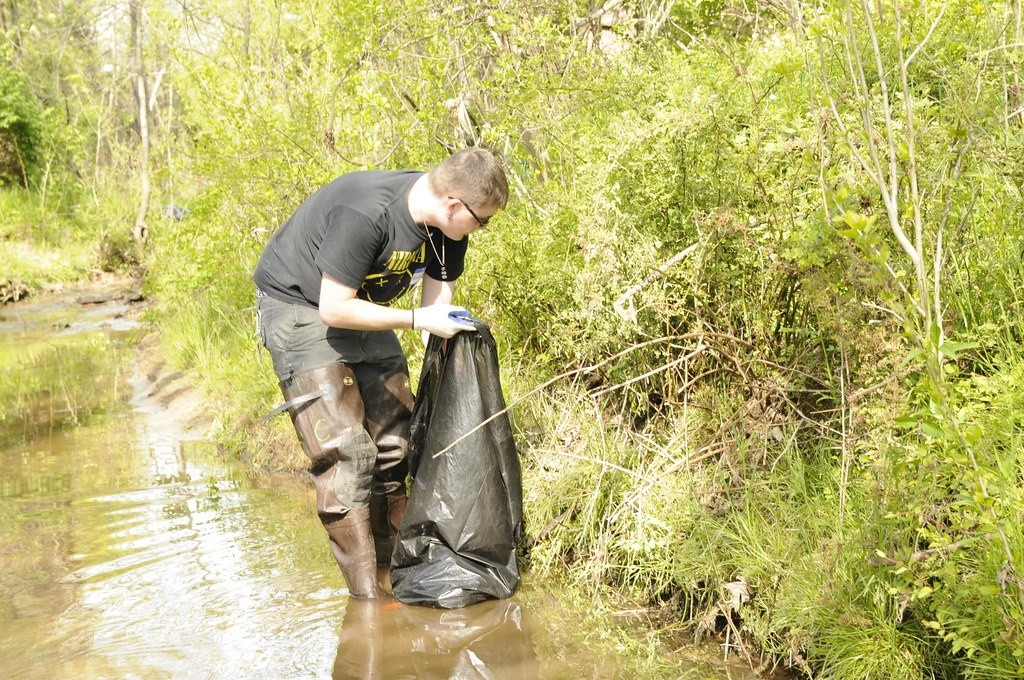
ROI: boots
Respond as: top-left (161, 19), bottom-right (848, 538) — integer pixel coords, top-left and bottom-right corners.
top-left (265, 362), bottom-right (392, 601)
top-left (348, 355), bottom-right (411, 564)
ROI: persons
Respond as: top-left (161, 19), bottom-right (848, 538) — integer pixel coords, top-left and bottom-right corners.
top-left (252, 146), bottom-right (509, 601)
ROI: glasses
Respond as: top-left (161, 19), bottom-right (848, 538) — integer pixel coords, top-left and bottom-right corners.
top-left (448, 196), bottom-right (489, 230)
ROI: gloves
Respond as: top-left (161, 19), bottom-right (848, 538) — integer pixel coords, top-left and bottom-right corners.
top-left (412, 304), bottom-right (482, 349)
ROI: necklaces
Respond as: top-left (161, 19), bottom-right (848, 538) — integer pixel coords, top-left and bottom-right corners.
top-left (414, 183), bottom-right (448, 280)
